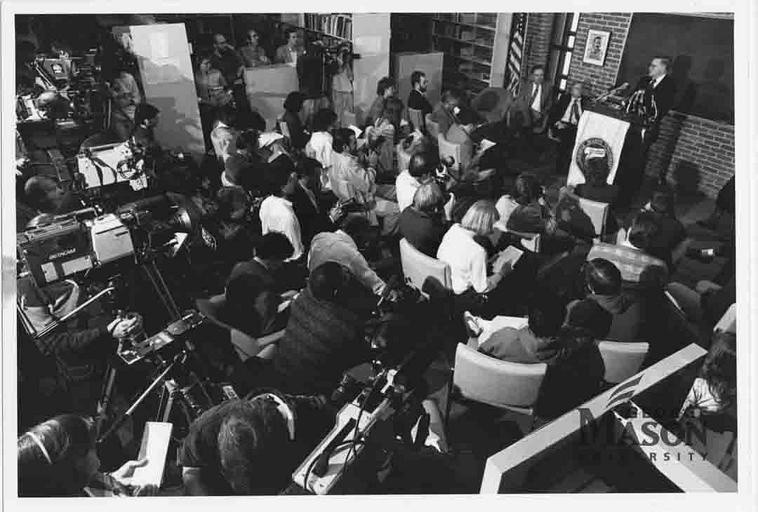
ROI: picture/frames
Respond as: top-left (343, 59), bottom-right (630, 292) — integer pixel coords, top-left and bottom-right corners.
top-left (582, 28), bottom-right (612, 67)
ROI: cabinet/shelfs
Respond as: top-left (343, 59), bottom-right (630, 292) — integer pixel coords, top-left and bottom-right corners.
top-left (111, 23), bottom-right (206, 155)
top-left (426, 14), bottom-right (517, 94)
top-left (269, 13), bottom-right (391, 125)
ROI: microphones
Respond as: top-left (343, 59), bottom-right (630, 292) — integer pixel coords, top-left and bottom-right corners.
top-left (599, 82), bottom-right (660, 122)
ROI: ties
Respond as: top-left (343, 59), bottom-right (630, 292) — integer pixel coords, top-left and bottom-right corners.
top-left (645, 78), bottom-right (655, 112)
top-left (529, 83), bottom-right (538, 108)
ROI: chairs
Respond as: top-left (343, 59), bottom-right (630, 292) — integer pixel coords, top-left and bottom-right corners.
top-left (16, 52), bottom-right (735, 496)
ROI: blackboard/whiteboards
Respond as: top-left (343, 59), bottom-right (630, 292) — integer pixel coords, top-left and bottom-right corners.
top-left (614, 11), bottom-right (735, 130)
